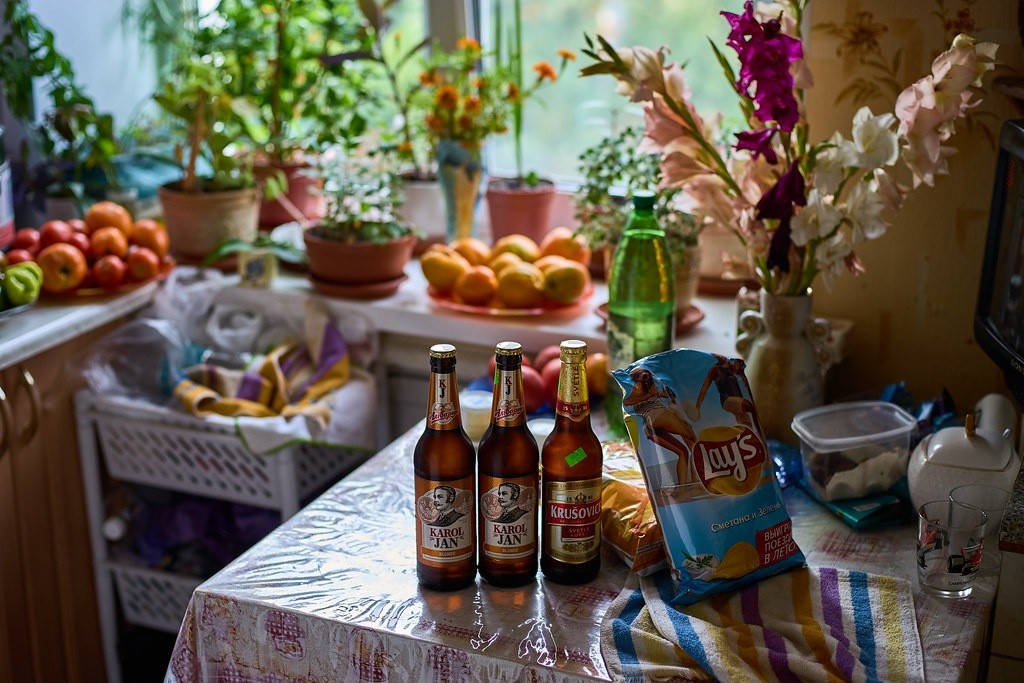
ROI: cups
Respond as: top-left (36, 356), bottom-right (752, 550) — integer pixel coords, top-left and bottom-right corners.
top-left (949, 484), bottom-right (1011, 577)
top-left (459, 390), bottom-right (493, 447)
top-left (240, 251), bottom-right (277, 290)
top-left (917, 501), bottom-right (987, 598)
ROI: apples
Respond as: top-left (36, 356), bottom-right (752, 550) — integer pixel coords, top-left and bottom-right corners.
top-left (489, 345), bottom-right (608, 410)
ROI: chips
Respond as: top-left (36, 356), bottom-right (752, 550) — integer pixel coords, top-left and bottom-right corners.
top-left (716, 543), bottom-right (759, 577)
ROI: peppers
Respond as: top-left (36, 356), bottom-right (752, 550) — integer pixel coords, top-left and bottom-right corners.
top-left (0, 261), bottom-right (43, 305)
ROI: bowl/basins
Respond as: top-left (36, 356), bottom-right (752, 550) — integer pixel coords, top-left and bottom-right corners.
top-left (792, 401), bottom-right (917, 501)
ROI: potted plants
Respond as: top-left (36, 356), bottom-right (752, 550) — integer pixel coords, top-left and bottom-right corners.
top-left (572, 123), bottom-right (733, 338)
top-left (482, 0), bottom-right (559, 252)
top-left (0, 0), bottom-right (419, 301)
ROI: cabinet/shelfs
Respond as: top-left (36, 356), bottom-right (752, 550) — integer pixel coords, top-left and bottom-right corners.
top-left (0, 299), bottom-right (156, 683)
top-left (74, 358), bottom-right (394, 683)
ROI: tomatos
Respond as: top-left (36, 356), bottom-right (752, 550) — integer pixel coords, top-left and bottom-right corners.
top-left (0, 202), bottom-right (167, 293)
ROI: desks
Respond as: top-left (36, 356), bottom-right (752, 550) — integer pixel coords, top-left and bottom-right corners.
top-left (163, 370), bottom-right (1003, 683)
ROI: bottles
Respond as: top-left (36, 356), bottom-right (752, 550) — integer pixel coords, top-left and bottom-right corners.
top-left (102, 481), bottom-right (149, 540)
top-left (414, 344), bottom-right (476, 591)
top-left (609, 190), bottom-right (675, 441)
top-left (541, 340), bottom-right (603, 585)
top-left (478, 341), bottom-right (539, 588)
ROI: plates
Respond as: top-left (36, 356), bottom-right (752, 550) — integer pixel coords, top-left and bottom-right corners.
top-left (428, 283), bottom-right (595, 315)
top-left (68, 262), bottom-right (176, 296)
top-left (270, 219), bottom-right (321, 263)
top-left (0, 299), bottom-right (37, 318)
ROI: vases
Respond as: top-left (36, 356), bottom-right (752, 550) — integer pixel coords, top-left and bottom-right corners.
top-left (745, 285), bottom-right (825, 447)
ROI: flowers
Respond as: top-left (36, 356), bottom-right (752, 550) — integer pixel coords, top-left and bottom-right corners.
top-left (398, 27), bottom-right (578, 163)
top-left (593, 0), bottom-right (1003, 298)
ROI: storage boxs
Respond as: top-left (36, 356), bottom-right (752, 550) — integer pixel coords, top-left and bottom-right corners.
top-left (792, 402), bottom-right (920, 504)
top-left (810, 314), bottom-right (858, 366)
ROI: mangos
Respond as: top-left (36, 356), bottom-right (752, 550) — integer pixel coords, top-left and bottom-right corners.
top-left (420, 226), bottom-right (591, 305)
